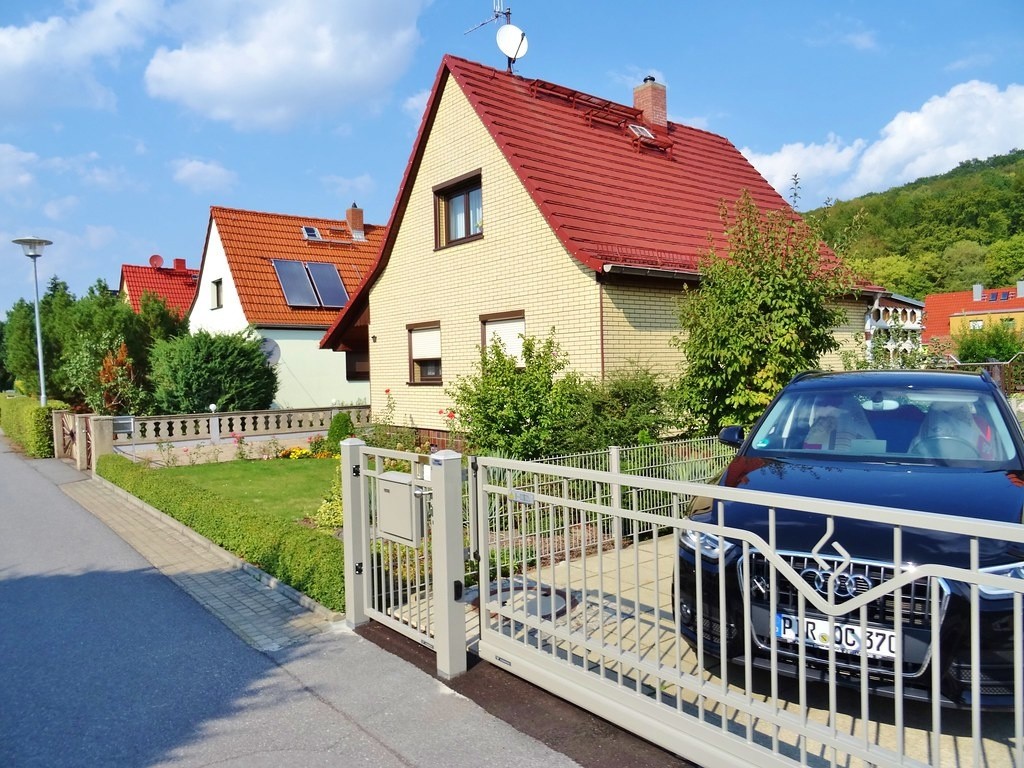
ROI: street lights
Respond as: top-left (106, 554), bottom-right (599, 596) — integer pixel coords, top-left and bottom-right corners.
top-left (12, 234), bottom-right (54, 411)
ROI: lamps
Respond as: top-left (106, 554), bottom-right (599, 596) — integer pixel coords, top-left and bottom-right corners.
top-left (371, 335), bottom-right (377, 343)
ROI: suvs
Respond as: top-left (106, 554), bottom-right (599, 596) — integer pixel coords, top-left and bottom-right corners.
top-left (668, 369), bottom-right (1024, 719)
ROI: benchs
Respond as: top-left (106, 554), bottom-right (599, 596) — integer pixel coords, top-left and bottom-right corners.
top-left (864, 404), bottom-right (926, 453)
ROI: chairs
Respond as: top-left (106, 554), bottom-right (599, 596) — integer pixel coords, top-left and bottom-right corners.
top-left (912, 401), bottom-right (986, 461)
top-left (801, 396), bottom-right (875, 455)
